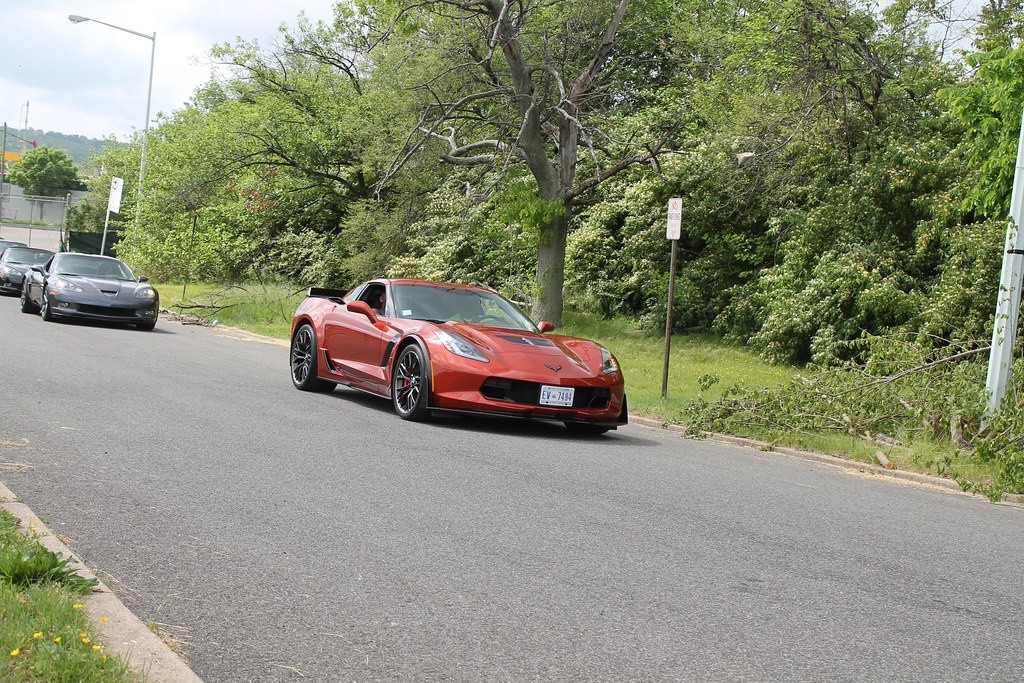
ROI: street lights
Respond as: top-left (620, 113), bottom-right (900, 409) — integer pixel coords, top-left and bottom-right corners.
top-left (69, 13), bottom-right (158, 228)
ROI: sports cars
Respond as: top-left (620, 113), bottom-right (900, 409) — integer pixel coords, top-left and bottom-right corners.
top-left (20, 252), bottom-right (160, 331)
top-left (289, 277), bottom-right (631, 437)
top-left (0, 247), bottom-right (55, 297)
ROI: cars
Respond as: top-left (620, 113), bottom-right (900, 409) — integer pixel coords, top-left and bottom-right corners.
top-left (0, 239), bottom-right (30, 258)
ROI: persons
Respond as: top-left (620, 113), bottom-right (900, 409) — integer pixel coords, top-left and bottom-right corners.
top-left (35, 254), bottom-right (46, 264)
top-left (372, 287), bottom-right (403, 316)
top-left (449, 294), bottom-right (507, 324)
top-left (97, 260), bottom-right (114, 278)
